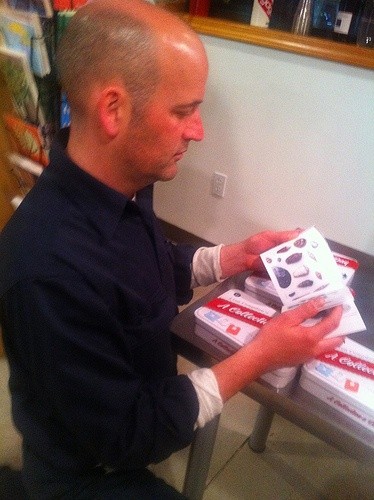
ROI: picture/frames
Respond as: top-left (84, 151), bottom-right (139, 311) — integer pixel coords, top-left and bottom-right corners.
top-left (56, 2), bottom-right (374, 73)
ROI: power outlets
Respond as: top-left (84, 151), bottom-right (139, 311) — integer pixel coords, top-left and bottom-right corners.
top-left (211, 171), bottom-right (227, 197)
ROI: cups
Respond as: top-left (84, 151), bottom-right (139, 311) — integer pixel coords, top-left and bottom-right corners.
top-left (250, 0), bottom-right (273, 28)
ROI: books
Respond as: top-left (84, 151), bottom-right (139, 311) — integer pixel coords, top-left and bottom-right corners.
top-left (260, 226), bottom-right (366, 340)
top-left (0, 0), bottom-right (73, 231)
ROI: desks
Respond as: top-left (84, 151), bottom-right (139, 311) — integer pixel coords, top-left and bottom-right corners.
top-left (168, 227), bottom-right (374, 499)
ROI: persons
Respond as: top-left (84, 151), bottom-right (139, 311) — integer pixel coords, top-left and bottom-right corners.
top-left (0, 0), bottom-right (345, 500)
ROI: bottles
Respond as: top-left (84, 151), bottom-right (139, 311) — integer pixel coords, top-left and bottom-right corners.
top-left (333, 0), bottom-right (361, 44)
top-left (291, 0), bottom-right (312, 37)
